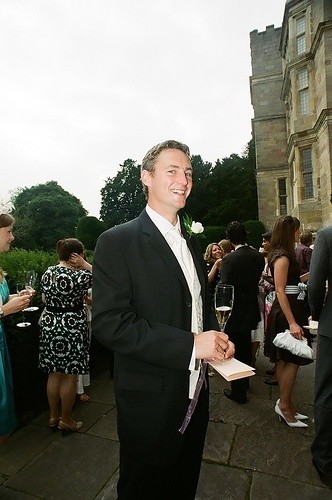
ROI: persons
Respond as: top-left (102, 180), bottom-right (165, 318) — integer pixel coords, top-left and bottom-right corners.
top-left (295, 230), bottom-right (318, 343)
top-left (204, 239), bottom-right (232, 377)
top-left (258, 232), bottom-right (279, 385)
top-left (263, 215), bottom-right (314, 427)
top-left (92, 139), bottom-right (235, 500)
top-left (307, 224), bottom-right (332, 486)
top-left (38, 238), bottom-right (92, 436)
top-left (0, 213), bottom-right (36, 436)
top-left (221, 221), bottom-right (265, 404)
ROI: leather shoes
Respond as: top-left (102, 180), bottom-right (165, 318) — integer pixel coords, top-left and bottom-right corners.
top-left (224, 389), bottom-right (232, 399)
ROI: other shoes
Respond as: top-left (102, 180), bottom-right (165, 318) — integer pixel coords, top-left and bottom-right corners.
top-left (208, 371), bottom-right (215, 377)
top-left (266, 368), bottom-right (276, 375)
top-left (263, 376), bottom-right (278, 385)
top-left (76, 393), bottom-right (91, 401)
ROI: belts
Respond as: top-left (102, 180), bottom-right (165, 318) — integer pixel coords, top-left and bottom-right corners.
top-left (46, 302), bottom-right (84, 313)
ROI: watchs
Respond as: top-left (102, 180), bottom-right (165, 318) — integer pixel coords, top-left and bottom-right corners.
top-left (0, 307), bottom-right (4, 318)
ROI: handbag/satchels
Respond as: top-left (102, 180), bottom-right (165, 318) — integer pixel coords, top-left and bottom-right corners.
top-left (273, 329), bottom-right (316, 360)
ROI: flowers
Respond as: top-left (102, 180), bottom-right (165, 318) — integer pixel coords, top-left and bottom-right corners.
top-left (182, 213), bottom-right (206, 240)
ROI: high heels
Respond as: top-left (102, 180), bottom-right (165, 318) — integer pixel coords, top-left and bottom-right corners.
top-left (275, 404), bottom-right (308, 427)
top-left (276, 398), bottom-right (308, 419)
top-left (58, 419), bottom-right (83, 437)
top-left (48, 417), bottom-right (61, 433)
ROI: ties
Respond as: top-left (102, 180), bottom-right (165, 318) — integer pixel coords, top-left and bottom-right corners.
top-left (171, 228), bottom-right (199, 335)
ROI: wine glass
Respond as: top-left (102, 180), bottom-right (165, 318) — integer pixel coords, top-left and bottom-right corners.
top-left (24, 270), bottom-right (39, 312)
top-left (214, 284), bottom-right (234, 332)
top-left (15, 282), bottom-right (31, 328)
